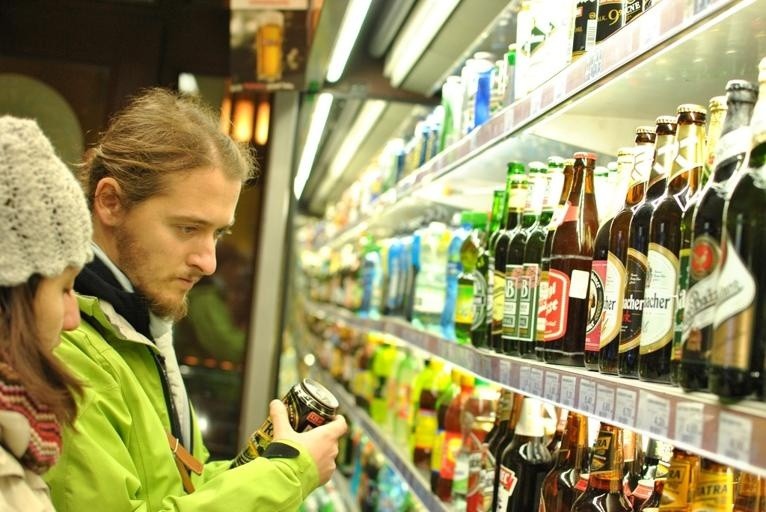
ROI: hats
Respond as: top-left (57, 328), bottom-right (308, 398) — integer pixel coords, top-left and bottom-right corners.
top-left (1, 114), bottom-right (92, 288)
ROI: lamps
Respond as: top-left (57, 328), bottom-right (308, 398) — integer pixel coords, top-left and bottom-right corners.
top-left (209, 73), bottom-right (273, 189)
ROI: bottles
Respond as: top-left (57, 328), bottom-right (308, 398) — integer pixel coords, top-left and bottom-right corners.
top-left (370, 1), bottom-right (766, 512)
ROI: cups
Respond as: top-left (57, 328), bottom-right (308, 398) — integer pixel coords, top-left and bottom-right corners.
top-left (256, 11), bottom-right (285, 84)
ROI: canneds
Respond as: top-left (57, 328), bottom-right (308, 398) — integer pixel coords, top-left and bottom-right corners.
top-left (466, 416), bottom-right (495, 512)
top-left (228, 378), bottom-right (339, 471)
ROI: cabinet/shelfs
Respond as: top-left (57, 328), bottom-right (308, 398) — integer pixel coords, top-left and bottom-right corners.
top-left (265, 0), bottom-right (764, 512)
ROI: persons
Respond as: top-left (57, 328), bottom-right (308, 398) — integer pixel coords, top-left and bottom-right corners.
top-left (174, 242), bottom-right (251, 402)
top-left (40, 84), bottom-right (349, 512)
top-left (0, 114), bottom-right (96, 512)
